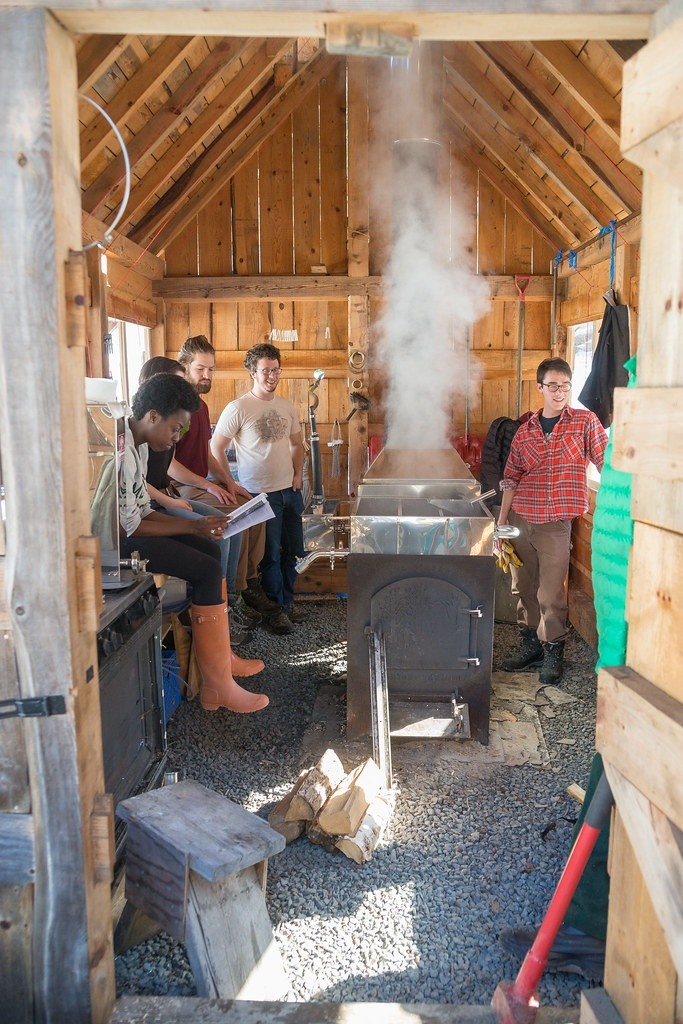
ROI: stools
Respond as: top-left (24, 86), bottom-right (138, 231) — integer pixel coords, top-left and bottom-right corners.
top-left (112, 779), bottom-right (288, 1000)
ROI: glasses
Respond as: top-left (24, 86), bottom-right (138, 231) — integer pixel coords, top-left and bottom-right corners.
top-left (539, 382), bottom-right (572, 392)
top-left (255, 368), bottom-right (283, 374)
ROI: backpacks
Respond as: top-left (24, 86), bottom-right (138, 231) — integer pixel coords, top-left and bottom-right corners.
top-left (479, 416), bottom-right (519, 506)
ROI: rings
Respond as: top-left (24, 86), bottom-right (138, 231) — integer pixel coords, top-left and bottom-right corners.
top-left (210, 529), bottom-right (216, 534)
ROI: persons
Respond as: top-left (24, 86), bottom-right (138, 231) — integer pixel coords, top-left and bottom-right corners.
top-left (210, 343), bottom-right (308, 633)
top-left (497, 356), bottom-right (609, 684)
top-left (117, 334), bottom-right (269, 713)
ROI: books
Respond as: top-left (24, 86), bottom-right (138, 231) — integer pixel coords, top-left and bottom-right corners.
top-left (223, 492), bottom-right (276, 540)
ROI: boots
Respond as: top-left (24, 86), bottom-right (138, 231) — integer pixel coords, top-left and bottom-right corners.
top-left (190, 600), bottom-right (269, 713)
top-left (501, 628), bottom-right (544, 671)
top-left (221, 577), bottom-right (264, 677)
top-left (538, 639), bottom-right (565, 683)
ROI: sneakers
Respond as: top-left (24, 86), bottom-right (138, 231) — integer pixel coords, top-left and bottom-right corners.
top-left (267, 612), bottom-right (295, 634)
top-left (229, 624), bottom-right (249, 645)
top-left (233, 596), bottom-right (263, 624)
top-left (246, 572), bottom-right (266, 598)
top-left (240, 588), bottom-right (277, 611)
top-left (284, 605), bottom-right (307, 622)
top-left (227, 603), bottom-right (253, 628)
